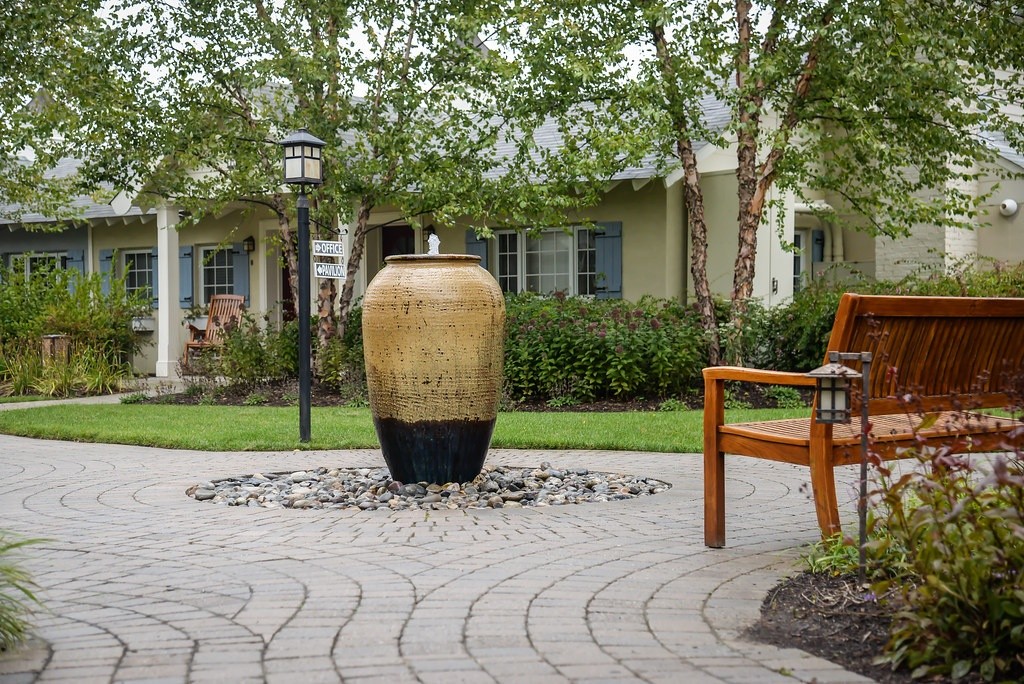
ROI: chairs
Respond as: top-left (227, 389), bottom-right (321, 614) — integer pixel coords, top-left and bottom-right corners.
top-left (181, 294), bottom-right (248, 382)
top-left (700, 291), bottom-right (1024, 555)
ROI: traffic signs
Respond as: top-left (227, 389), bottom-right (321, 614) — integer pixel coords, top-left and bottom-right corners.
top-left (312, 240), bottom-right (345, 256)
top-left (314, 262), bottom-right (346, 279)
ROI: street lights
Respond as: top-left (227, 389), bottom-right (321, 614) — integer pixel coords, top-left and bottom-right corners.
top-left (277, 127), bottom-right (331, 444)
